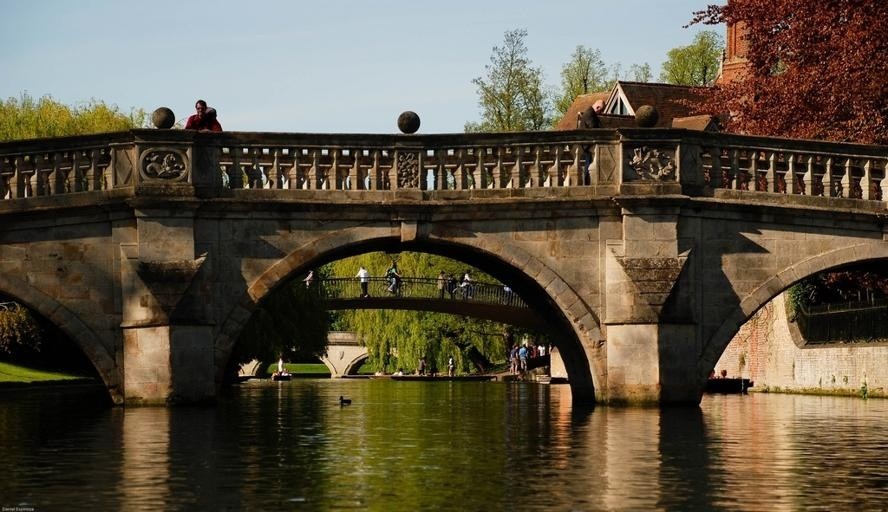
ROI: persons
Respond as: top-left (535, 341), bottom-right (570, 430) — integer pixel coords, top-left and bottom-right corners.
top-left (398, 368), bottom-right (404, 376)
top-left (418, 357), bottom-right (428, 376)
top-left (281, 368), bottom-right (292, 376)
top-left (448, 354), bottom-right (454, 377)
top-left (185, 100), bottom-right (222, 131)
top-left (355, 267), bottom-right (370, 297)
top-left (437, 270), bottom-right (474, 299)
top-left (509, 344), bottom-right (546, 376)
top-left (580, 99), bottom-right (606, 185)
top-left (385, 264), bottom-right (401, 293)
top-left (272, 370), bottom-right (280, 376)
top-left (303, 271), bottom-right (314, 288)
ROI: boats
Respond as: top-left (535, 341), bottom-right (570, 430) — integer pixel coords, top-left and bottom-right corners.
top-left (271, 369), bottom-right (293, 382)
top-left (388, 374), bottom-right (497, 382)
top-left (704, 375), bottom-right (753, 394)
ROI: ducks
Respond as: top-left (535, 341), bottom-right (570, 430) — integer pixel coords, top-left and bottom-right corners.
top-left (338, 396), bottom-right (352, 404)
